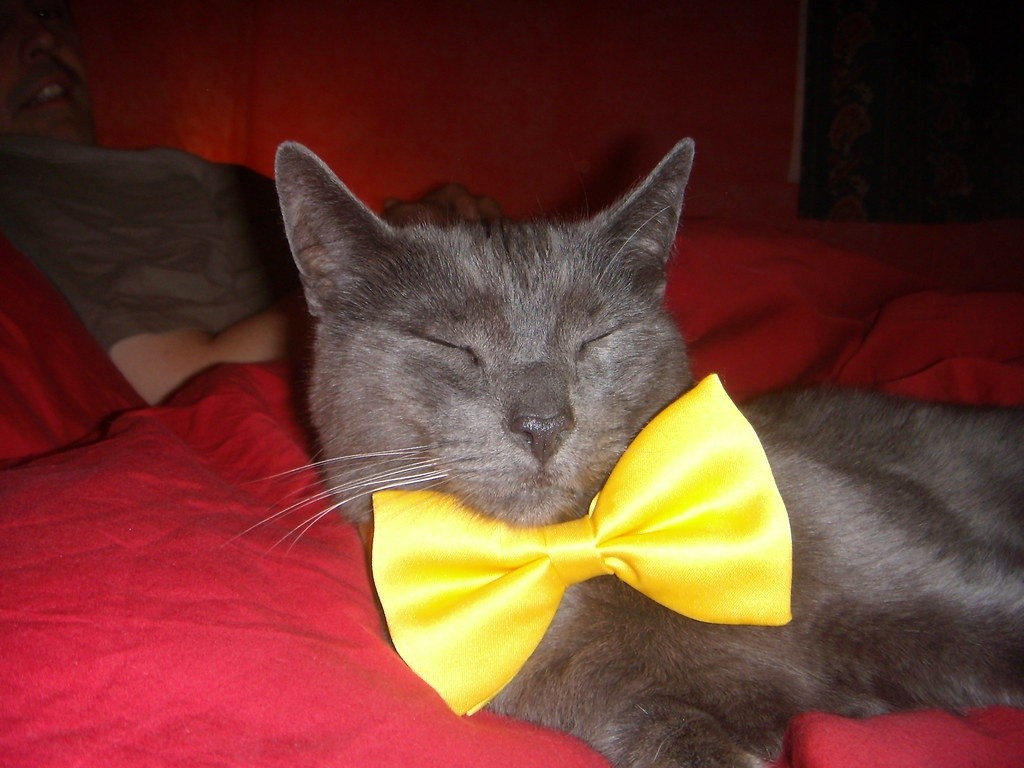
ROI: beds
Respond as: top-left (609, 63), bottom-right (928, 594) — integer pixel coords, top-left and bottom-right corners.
top-left (3, 131), bottom-right (1024, 768)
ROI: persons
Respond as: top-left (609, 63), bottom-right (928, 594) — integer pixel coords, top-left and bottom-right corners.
top-left (0, 0), bottom-right (515, 413)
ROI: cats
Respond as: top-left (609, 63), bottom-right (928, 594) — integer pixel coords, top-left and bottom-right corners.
top-left (273, 141), bottom-right (1023, 768)
